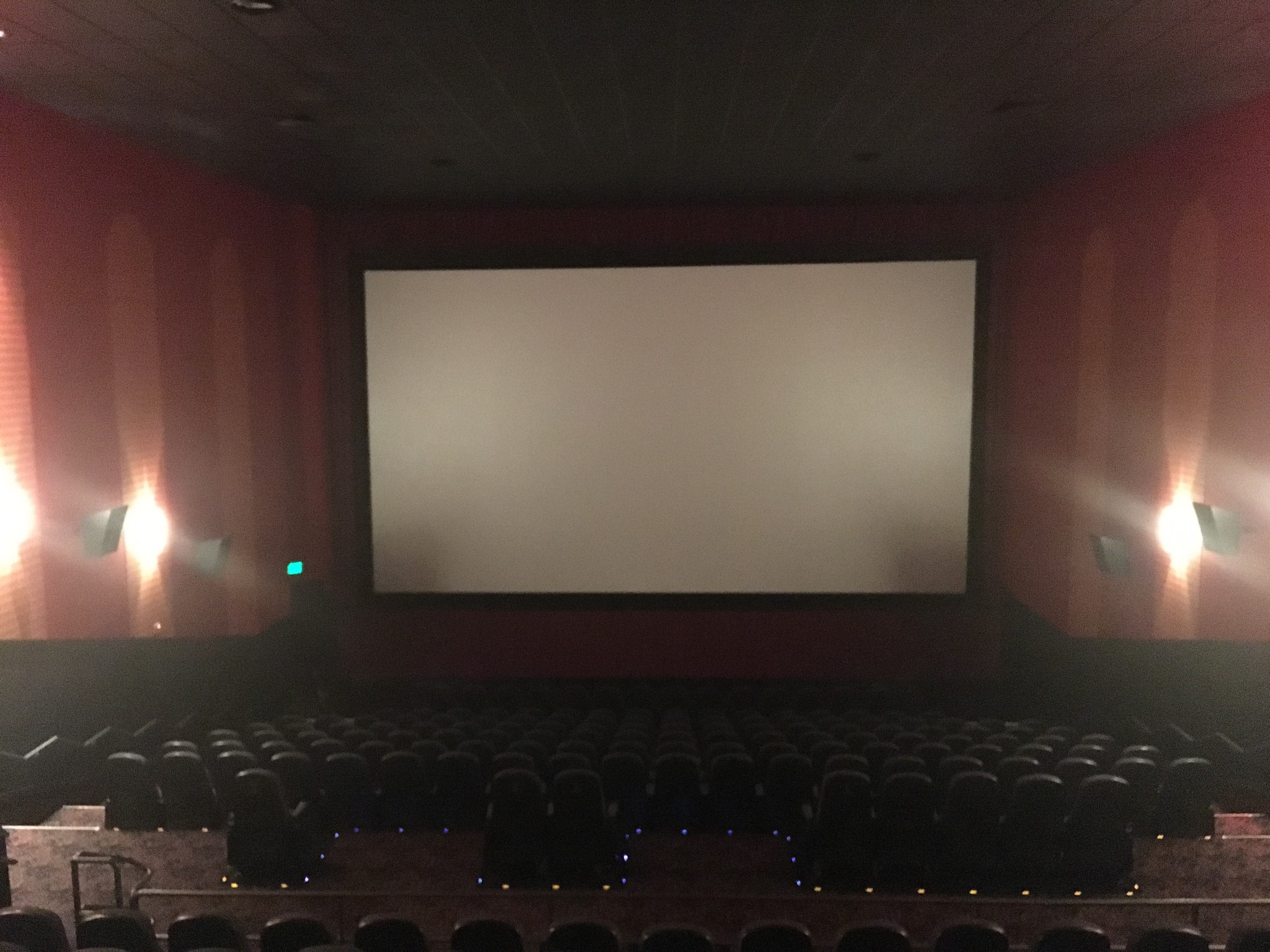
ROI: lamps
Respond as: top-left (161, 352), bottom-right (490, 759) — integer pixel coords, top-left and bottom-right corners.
top-left (80, 505), bottom-right (127, 558)
top-left (1191, 500), bottom-right (1242, 558)
top-left (196, 535), bottom-right (233, 580)
top-left (1088, 532), bottom-right (1131, 576)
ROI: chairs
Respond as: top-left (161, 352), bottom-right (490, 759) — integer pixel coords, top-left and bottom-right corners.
top-left (0, 705), bottom-right (1270, 952)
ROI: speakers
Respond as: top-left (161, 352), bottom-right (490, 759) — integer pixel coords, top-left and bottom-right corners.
top-left (197, 537), bottom-right (230, 576)
top-left (84, 504), bottom-right (126, 555)
top-left (1091, 534), bottom-right (1126, 573)
top-left (1195, 501), bottom-right (1238, 555)
